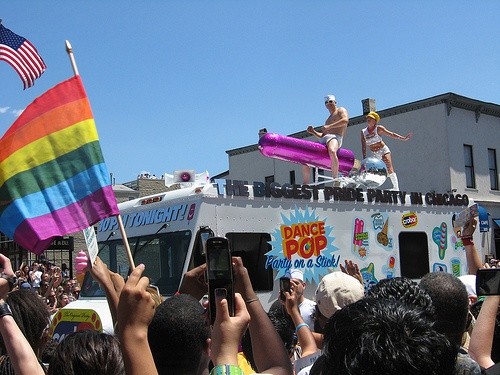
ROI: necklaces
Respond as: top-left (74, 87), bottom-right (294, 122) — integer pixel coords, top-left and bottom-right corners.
top-left (297, 301), bottom-right (303, 307)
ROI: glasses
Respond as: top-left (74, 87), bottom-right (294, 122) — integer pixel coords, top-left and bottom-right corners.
top-left (59, 291), bottom-right (65, 293)
top-left (325, 100), bottom-right (333, 105)
top-left (43, 283), bottom-right (49, 286)
top-left (148, 284), bottom-right (162, 305)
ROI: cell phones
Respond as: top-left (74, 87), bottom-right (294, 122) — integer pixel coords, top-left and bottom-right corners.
top-left (280, 277), bottom-right (291, 300)
top-left (476, 268), bottom-right (500, 297)
top-left (206, 236), bottom-right (235, 326)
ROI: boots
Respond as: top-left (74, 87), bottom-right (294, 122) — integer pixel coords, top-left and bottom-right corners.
top-left (387, 171), bottom-right (400, 191)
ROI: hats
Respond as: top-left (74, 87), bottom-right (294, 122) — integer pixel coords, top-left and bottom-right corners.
top-left (366, 112), bottom-right (380, 123)
top-left (21, 283), bottom-right (31, 289)
top-left (290, 270), bottom-right (303, 282)
top-left (324, 95), bottom-right (335, 103)
top-left (458, 275), bottom-right (478, 297)
top-left (57, 286), bottom-right (64, 291)
top-left (315, 271), bottom-right (365, 319)
top-left (490, 259), bottom-right (499, 263)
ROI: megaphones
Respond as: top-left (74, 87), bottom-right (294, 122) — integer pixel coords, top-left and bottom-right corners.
top-left (164, 170), bottom-right (209, 187)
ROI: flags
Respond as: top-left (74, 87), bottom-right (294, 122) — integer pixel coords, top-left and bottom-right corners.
top-left (0, 74), bottom-right (119, 254)
top-left (0, 21), bottom-right (48, 91)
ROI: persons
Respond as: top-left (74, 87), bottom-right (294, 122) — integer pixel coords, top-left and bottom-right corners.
top-left (359, 112), bottom-right (410, 190)
top-left (302, 94), bottom-right (349, 188)
top-left (0, 221), bottom-right (500, 375)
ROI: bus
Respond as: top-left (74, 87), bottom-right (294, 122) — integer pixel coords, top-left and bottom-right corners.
top-left (41, 183), bottom-right (496, 363)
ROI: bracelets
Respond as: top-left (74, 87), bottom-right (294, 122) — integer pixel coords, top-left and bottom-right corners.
top-left (0, 303), bottom-right (14, 318)
top-left (295, 323), bottom-right (309, 332)
top-left (209, 364), bottom-right (244, 375)
top-left (245, 298), bottom-right (259, 303)
top-left (463, 236), bottom-right (473, 239)
